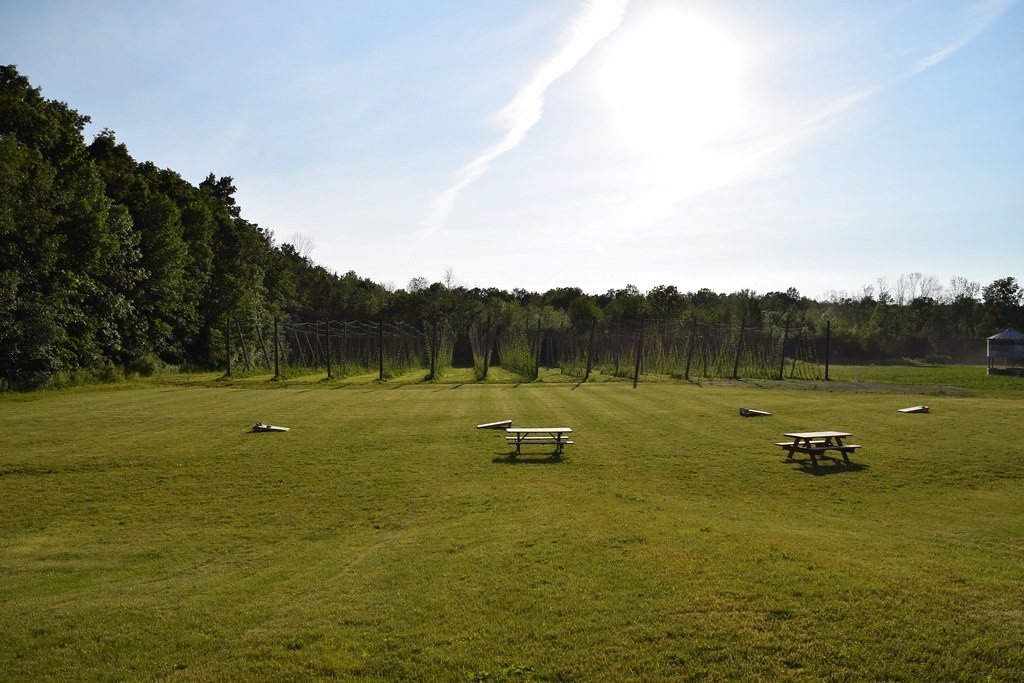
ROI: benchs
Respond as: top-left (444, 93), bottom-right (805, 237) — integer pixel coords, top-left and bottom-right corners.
top-left (506, 440), bottom-right (574, 458)
top-left (505, 436), bottom-right (569, 454)
top-left (775, 439), bottom-right (831, 459)
top-left (805, 445), bottom-right (862, 466)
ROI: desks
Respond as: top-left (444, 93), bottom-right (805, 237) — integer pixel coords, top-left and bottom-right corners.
top-left (784, 431), bottom-right (851, 468)
top-left (506, 427), bottom-right (572, 458)
